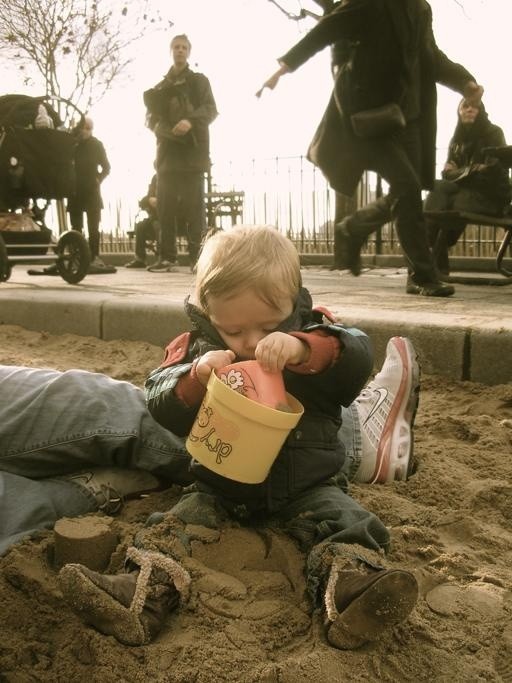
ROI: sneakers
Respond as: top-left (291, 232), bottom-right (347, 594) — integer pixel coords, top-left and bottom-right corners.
top-left (406, 273), bottom-right (455, 297)
top-left (125, 260), bottom-right (144, 268)
top-left (355, 335), bottom-right (421, 483)
top-left (339, 216), bottom-right (364, 275)
top-left (147, 259), bottom-right (178, 272)
top-left (64, 468), bottom-right (169, 515)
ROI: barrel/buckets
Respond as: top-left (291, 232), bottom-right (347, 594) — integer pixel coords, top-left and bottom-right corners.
top-left (185, 367), bottom-right (304, 484)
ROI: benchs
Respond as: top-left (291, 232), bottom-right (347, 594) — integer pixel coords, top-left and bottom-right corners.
top-left (126, 188), bottom-right (244, 273)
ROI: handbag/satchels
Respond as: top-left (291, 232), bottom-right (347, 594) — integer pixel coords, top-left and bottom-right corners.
top-left (350, 104), bottom-right (406, 139)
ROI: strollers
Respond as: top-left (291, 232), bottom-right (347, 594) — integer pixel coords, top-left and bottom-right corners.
top-left (0, 94), bottom-right (91, 284)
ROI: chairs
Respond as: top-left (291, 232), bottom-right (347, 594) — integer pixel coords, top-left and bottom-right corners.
top-left (419, 145), bottom-right (512, 286)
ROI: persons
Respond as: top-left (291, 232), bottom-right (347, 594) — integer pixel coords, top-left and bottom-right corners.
top-left (423, 94), bottom-right (512, 277)
top-left (63, 117), bottom-right (111, 273)
top-left (144, 33), bottom-right (220, 273)
top-left (0, 334), bottom-right (420, 559)
top-left (58, 223), bottom-right (420, 650)
top-left (257, 0), bottom-right (483, 296)
top-left (123, 159), bottom-right (186, 268)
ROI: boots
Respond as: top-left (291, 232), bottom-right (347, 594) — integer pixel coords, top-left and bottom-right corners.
top-left (61, 546), bottom-right (191, 647)
top-left (310, 544), bottom-right (418, 650)
